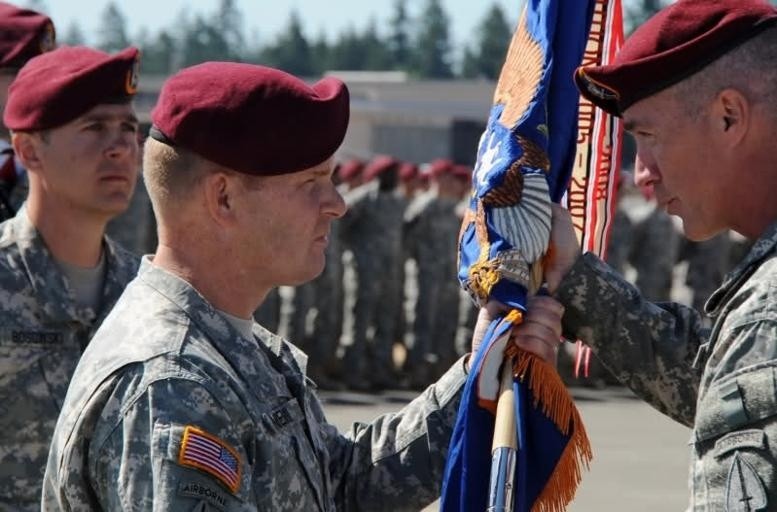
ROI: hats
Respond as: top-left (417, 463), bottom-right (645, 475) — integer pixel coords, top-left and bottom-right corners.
top-left (569, 0), bottom-right (777, 120)
top-left (0, 44), bottom-right (140, 134)
top-left (148, 59), bottom-right (357, 175)
top-left (0, 1), bottom-right (60, 70)
top-left (330, 149), bottom-right (472, 186)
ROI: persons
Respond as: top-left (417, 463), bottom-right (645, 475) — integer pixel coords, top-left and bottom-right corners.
top-left (547, 0), bottom-right (777, 512)
top-left (41, 60), bottom-right (568, 512)
top-left (256, 157), bottom-right (755, 397)
top-left (0, 1), bottom-right (162, 511)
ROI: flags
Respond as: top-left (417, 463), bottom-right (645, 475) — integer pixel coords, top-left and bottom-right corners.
top-left (440, 0), bottom-right (627, 512)
